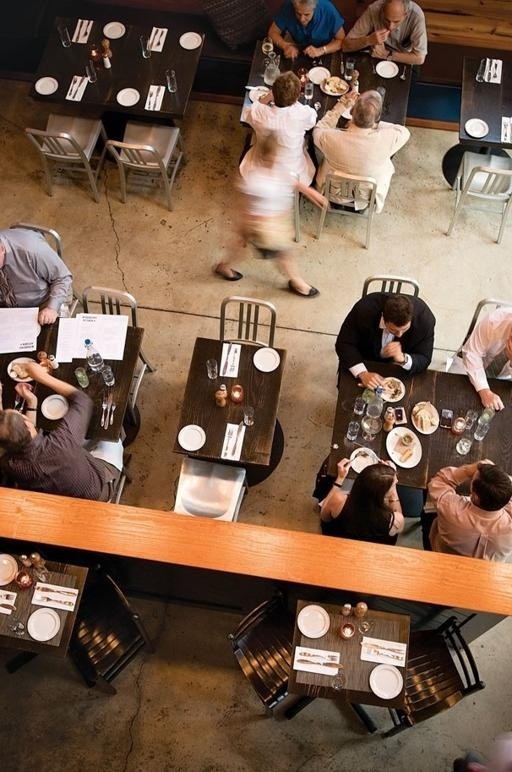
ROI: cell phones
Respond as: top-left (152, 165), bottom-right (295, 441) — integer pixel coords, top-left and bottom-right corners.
top-left (440, 409), bottom-right (453, 429)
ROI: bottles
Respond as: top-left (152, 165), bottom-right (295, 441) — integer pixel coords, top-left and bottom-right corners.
top-left (83, 339), bottom-right (105, 375)
top-left (46, 354), bottom-right (59, 369)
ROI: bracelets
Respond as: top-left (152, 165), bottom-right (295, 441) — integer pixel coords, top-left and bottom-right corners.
top-left (323, 46), bottom-right (327, 55)
top-left (26, 407), bottom-right (37, 411)
top-left (389, 500), bottom-right (399, 502)
top-left (387, 50), bottom-right (392, 58)
top-left (334, 482), bottom-right (342, 488)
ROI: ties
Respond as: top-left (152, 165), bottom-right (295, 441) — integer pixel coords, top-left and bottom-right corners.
top-left (0, 269), bottom-right (18, 307)
top-left (485, 349), bottom-right (509, 377)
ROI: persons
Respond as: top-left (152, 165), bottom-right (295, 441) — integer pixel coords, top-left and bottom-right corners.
top-left (312, 91), bottom-right (410, 215)
top-left (317, 457), bottom-right (405, 546)
top-left (334, 292), bottom-right (436, 390)
top-left (420, 458), bottom-right (512, 564)
top-left (214, 131), bottom-right (330, 298)
top-left (267, 0), bottom-right (345, 60)
top-left (0, 363), bottom-right (124, 503)
top-left (0, 228), bottom-right (73, 326)
top-left (445, 307), bottom-right (512, 411)
top-left (341, 0), bottom-right (428, 95)
top-left (245, 71), bottom-right (319, 188)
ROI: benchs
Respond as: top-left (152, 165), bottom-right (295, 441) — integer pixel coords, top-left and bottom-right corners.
top-left (0, 0), bottom-right (512, 133)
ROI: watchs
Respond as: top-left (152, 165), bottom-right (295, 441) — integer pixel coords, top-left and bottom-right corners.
top-left (400, 353), bottom-right (409, 365)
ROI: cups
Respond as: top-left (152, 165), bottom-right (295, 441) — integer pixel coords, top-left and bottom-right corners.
top-left (340, 622), bottom-right (356, 641)
top-left (165, 68), bottom-right (178, 93)
top-left (332, 673), bottom-right (347, 691)
top-left (56, 23), bottom-right (72, 47)
top-left (346, 421), bottom-right (361, 441)
top-left (358, 618), bottom-right (376, 635)
top-left (206, 358), bottom-right (218, 380)
top-left (243, 406), bottom-right (255, 426)
top-left (86, 42), bottom-right (100, 60)
top-left (83, 59), bottom-right (97, 83)
top-left (100, 365), bottom-right (115, 387)
top-left (475, 58), bottom-right (491, 83)
top-left (353, 394), bottom-right (367, 415)
top-left (14, 569), bottom-right (34, 589)
top-left (344, 57), bottom-right (356, 81)
top-left (304, 78), bottom-right (314, 100)
top-left (230, 384), bottom-right (244, 403)
top-left (74, 367), bottom-right (90, 389)
top-left (139, 34), bottom-right (152, 59)
top-left (451, 407), bottom-right (496, 455)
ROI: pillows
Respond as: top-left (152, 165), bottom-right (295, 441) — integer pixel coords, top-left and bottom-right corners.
top-left (201, 0), bottom-right (269, 49)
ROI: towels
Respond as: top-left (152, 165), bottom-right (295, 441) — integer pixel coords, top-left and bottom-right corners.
top-left (476, 57), bottom-right (503, 85)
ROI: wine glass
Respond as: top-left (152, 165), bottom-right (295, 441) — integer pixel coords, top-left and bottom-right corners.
top-left (261, 37), bottom-right (274, 66)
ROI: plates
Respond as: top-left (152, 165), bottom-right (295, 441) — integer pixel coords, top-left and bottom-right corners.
top-left (7, 357), bottom-right (38, 383)
top-left (385, 426), bottom-right (423, 469)
top-left (307, 66), bottom-right (331, 85)
top-left (464, 118), bottom-right (489, 139)
top-left (350, 446), bottom-right (378, 474)
top-left (249, 85), bottom-right (272, 104)
top-left (116, 88), bottom-right (140, 107)
top-left (102, 21), bottom-right (126, 40)
top-left (375, 60), bottom-right (400, 79)
top-left (0, 554), bottom-right (19, 586)
top-left (297, 604), bottom-right (331, 639)
top-left (376, 376), bottom-right (406, 403)
top-left (252, 346), bottom-right (281, 373)
top-left (410, 401), bottom-right (440, 435)
top-left (26, 607), bottom-right (60, 642)
top-left (40, 394), bottom-right (70, 421)
top-left (368, 664), bottom-right (404, 700)
top-left (34, 76), bottom-right (59, 96)
top-left (179, 31), bottom-right (202, 50)
top-left (177, 423), bottom-right (207, 453)
top-left (319, 76), bottom-right (350, 96)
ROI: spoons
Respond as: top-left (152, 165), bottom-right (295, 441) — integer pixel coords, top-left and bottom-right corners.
top-left (109, 401), bottom-right (118, 426)
top-left (400, 65), bottom-right (408, 81)
top-left (314, 101), bottom-right (322, 119)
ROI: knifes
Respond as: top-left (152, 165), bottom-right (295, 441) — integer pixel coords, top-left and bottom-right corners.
top-left (221, 341), bottom-right (232, 376)
top-left (153, 83), bottom-right (164, 111)
top-left (486, 58), bottom-right (493, 82)
top-left (360, 640), bottom-right (405, 655)
top-left (34, 585), bottom-right (77, 596)
top-left (148, 27), bottom-right (158, 49)
top-left (75, 19), bottom-right (84, 42)
top-left (231, 420), bottom-right (243, 457)
top-left (104, 392), bottom-right (113, 430)
top-left (297, 658), bottom-right (343, 670)
top-left (0, 603), bottom-right (17, 611)
top-left (73, 73), bottom-right (88, 99)
top-left (367, 445), bottom-right (390, 466)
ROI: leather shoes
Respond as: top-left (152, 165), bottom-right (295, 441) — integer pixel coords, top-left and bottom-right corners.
top-left (216, 264), bottom-right (242, 281)
top-left (289, 280), bottom-right (318, 296)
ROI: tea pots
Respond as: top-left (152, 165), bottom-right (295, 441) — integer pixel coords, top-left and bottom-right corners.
top-left (264, 52), bottom-right (281, 87)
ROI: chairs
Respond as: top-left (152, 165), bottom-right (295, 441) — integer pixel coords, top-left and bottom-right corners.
top-left (227, 597), bottom-right (302, 721)
top-left (316, 171), bottom-right (376, 251)
top-left (381, 611), bottom-right (488, 738)
top-left (445, 296), bottom-right (511, 374)
top-left (162, 458), bottom-right (252, 526)
top-left (105, 121), bottom-right (187, 213)
top-left (244, 174), bottom-right (300, 249)
top-left (25, 113), bottom-right (116, 204)
top-left (359, 273), bottom-right (424, 300)
top-left (2, 224), bottom-right (67, 308)
top-left (69, 563), bottom-right (152, 697)
top-left (216, 293), bottom-right (282, 352)
top-left (444, 151), bottom-right (512, 244)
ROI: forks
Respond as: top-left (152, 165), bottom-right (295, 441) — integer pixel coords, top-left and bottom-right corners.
top-left (0, 593), bottom-right (15, 600)
top-left (299, 651), bottom-right (338, 661)
top-left (365, 648), bottom-right (403, 661)
top-left (68, 76), bottom-right (77, 96)
top-left (100, 397), bottom-right (108, 427)
top-left (148, 86), bottom-right (154, 110)
top-left (83, 19), bottom-right (93, 38)
top-left (157, 30), bottom-right (166, 48)
top-left (493, 61), bottom-right (500, 78)
top-left (12, 393), bottom-right (20, 410)
top-left (223, 428), bottom-right (233, 457)
top-left (345, 450), bottom-right (364, 468)
top-left (33, 596), bottom-right (74, 606)
top-left (229, 348), bottom-right (238, 375)
